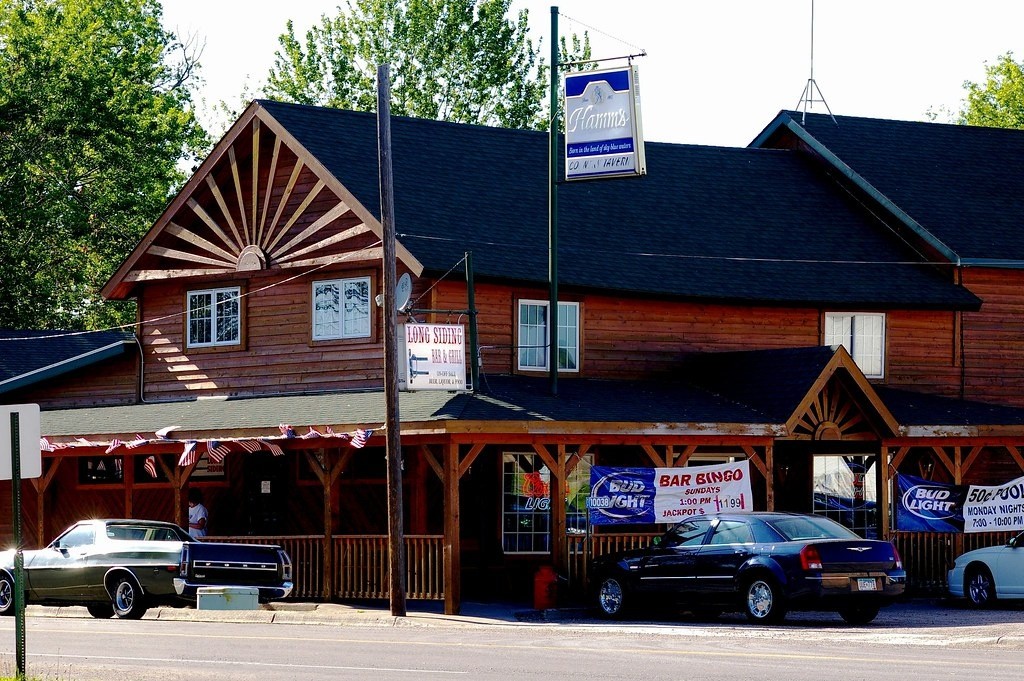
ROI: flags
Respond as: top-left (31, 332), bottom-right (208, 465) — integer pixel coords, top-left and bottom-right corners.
top-left (257, 438), bottom-right (285, 456)
top-left (155, 426), bottom-right (179, 439)
top-left (350, 428), bottom-right (374, 448)
top-left (234, 438), bottom-right (261, 453)
top-left (207, 440), bottom-right (231, 462)
top-left (40, 436), bottom-right (95, 452)
top-left (303, 426), bottom-right (321, 440)
top-left (279, 424), bottom-right (295, 438)
top-left (178, 440), bottom-right (198, 466)
top-left (326, 426), bottom-right (348, 440)
top-left (127, 432), bottom-right (150, 449)
top-left (105, 439), bottom-right (121, 454)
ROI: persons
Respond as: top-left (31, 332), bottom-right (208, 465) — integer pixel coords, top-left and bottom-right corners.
top-left (188, 487), bottom-right (208, 535)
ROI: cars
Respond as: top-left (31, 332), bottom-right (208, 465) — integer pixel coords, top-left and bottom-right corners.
top-left (589, 510), bottom-right (906, 627)
top-left (948, 531), bottom-right (1024, 611)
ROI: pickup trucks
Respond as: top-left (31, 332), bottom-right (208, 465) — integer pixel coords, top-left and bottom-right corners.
top-left (0, 518), bottom-right (295, 621)
